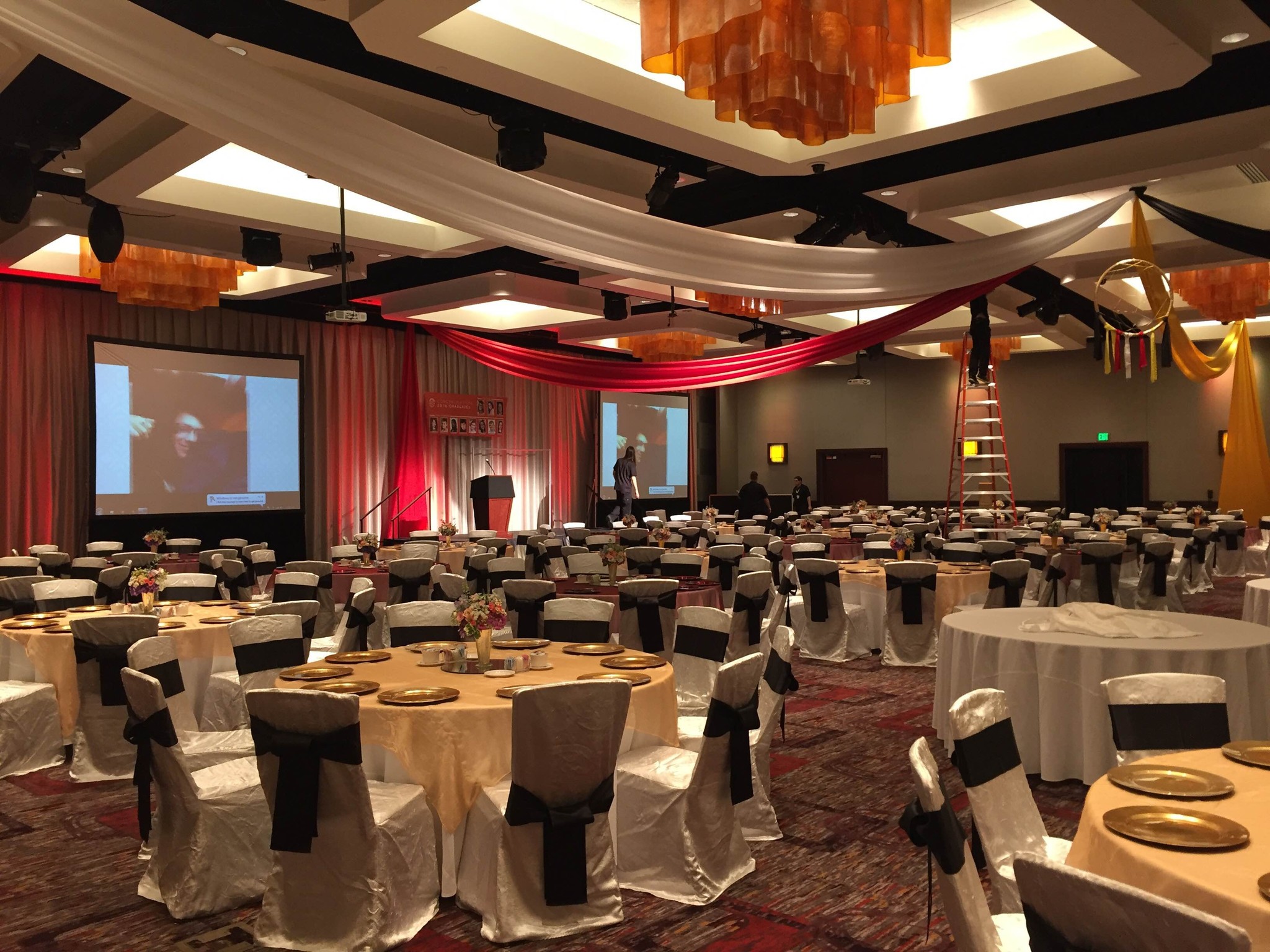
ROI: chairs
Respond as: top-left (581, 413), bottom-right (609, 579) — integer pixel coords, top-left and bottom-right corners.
top-left (947, 687), bottom-right (1073, 914)
top-left (0, 679), bottom-right (65, 780)
top-left (68, 615), bottom-right (161, 783)
top-left (126, 636), bottom-right (256, 772)
top-left (614, 652), bottom-right (765, 906)
top-left (909, 736), bottom-right (1031, 952)
top-left (1013, 853), bottom-right (1250, 952)
top-left (1100, 672), bottom-right (1231, 767)
top-left (454, 679), bottom-right (633, 944)
top-left (226, 614), bottom-right (307, 709)
top-left (119, 668), bottom-right (274, 918)
top-left (0, 505), bottom-right (1270, 668)
top-left (245, 687), bottom-right (440, 952)
top-left (200, 601), bottom-right (321, 731)
top-left (678, 626), bottom-right (799, 841)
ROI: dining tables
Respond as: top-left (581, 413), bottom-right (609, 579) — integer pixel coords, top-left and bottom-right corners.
top-left (1063, 747), bottom-right (1270, 952)
top-left (0, 600), bottom-right (269, 747)
top-left (931, 608), bottom-right (1270, 785)
top-left (272, 641), bottom-right (678, 899)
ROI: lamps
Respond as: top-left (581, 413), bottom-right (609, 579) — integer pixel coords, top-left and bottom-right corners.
top-left (1218, 430), bottom-right (1228, 456)
top-left (767, 443), bottom-right (788, 464)
top-left (958, 437), bottom-right (982, 461)
top-left (1, 1), bottom-right (1270, 371)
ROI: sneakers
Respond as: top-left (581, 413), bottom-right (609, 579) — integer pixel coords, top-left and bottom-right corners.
top-left (606, 515), bottom-right (613, 529)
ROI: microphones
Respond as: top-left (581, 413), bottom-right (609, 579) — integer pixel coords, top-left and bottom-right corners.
top-left (485, 458), bottom-right (495, 475)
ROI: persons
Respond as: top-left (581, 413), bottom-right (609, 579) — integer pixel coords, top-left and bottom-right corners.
top-left (477, 399), bottom-right (503, 415)
top-left (430, 417), bottom-right (503, 434)
top-left (792, 476), bottom-right (812, 515)
top-left (738, 471), bottom-right (771, 520)
top-left (606, 446), bottom-right (639, 530)
top-left (617, 429), bottom-right (648, 462)
top-left (129, 405), bottom-right (208, 492)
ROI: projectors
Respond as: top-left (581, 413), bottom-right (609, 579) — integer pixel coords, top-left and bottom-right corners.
top-left (325, 310), bottom-right (367, 324)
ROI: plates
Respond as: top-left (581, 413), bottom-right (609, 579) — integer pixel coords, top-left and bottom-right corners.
top-left (332, 561), bottom-right (392, 574)
top-left (823, 525), bottom-right (902, 535)
top-left (277, 565), bottom-right (287, 571)
top-left (279, 637), bottom-right (667, 706)
top-left (686, 547), bottom-right (704, 551)
top-left (549, 571), bottom-right (721, 594)
top-left (1, 596), bottom-right (278, 636)
top-left (1100, 718), bottom-right (1270, 915)
top-left (830, 557), bottom-right (992, 576)
